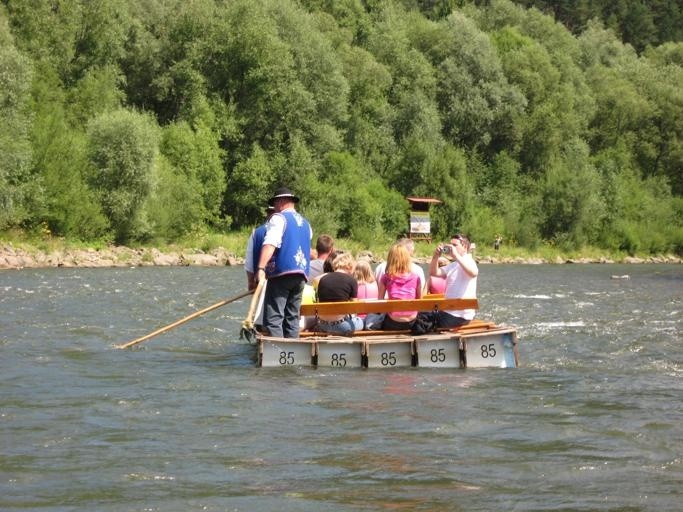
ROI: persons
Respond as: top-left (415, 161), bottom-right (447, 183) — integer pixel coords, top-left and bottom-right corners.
top-left (302, 234), bottom-right (335, 286)
top-left (372, 237), bottom-right (424, 301)
top-left (245, 203), bottom-right (276, 296)
top-left (309, 249), bottom-right (342, 292)
top-left (429, 231), bottom-right (480, 330)
top-left (347, 261), bottom-right (381, 322)
top-left (493, 236), bottom-right (500, 250)
top-left (372, 244), bottom-right (424, 332)
top-left (423, 255), bottom-right (452, 294)
top-left (250, 186), bottom-right (314, 339)
top-left (310, 253), bottom-right (365, 334)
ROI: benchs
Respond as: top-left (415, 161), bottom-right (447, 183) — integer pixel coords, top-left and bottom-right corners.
top-left (298, 294), bottom-right (494, 338)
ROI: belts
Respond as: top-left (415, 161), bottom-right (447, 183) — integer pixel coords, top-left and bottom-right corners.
top-left (316, 318), bottom-right (346, 326)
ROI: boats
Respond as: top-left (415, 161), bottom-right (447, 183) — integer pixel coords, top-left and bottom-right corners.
top-left (252, 297), bottom-right (520, 372)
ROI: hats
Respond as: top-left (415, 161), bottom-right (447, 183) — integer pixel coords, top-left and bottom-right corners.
top-left (265, 205), bottom-right (275, 211)
top-left (268, 187), bottom-right (299, 206)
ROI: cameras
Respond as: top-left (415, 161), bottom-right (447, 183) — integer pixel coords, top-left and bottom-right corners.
top-left (441, 246), bottom-right (451, 253)
